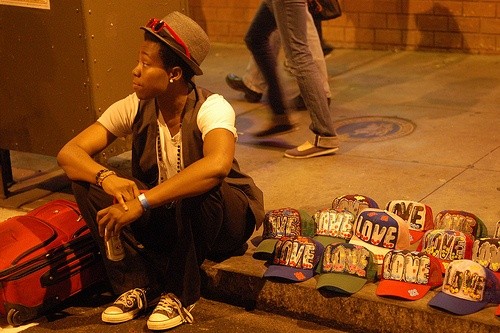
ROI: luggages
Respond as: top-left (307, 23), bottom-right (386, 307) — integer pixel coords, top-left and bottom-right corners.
top-left (0, 199), bottom-right (107, 327)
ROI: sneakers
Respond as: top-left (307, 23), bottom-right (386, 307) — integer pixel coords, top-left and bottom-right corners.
top-left (101, 287), bottom-right (168, 323)
top-left (284, 140), bottom-right (340, 158)
top-left (253, 123), bottom-right (298, 139)
top-left (147, 292), bottom-right (198, 330)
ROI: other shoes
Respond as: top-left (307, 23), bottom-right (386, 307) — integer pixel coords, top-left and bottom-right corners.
top-left (225, 72), bottom-right (263, 103)
top-left (323, 44), bottom-right (334, 59)
top-left (289, 95), bottom-right (332, 111)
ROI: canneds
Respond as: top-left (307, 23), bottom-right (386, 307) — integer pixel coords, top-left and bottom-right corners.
top-left (104, 225), bottom-right (126, 262)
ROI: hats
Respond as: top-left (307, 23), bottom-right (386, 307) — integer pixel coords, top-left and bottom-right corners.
top-left (492, 221), bottom-right (500, 239)
top-left (428, 259), bottom-right (497, 316)
top-left (494, 304), bottom-right (500, 320)
top-left (375, 249), bottom-right (445, 303)
top-left (488, 270), bottom-right (500, 304)
top-left (140, 11), bottom-right (211, 76)
top-left (253, 194), bottom-right (484, 257)
top-left (263, 234), bottom-right (324, 282)
top-left (316, 242), bottom-right (379, 293)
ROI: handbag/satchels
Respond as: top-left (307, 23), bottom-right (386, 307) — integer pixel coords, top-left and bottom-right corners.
top-left (308, 0), bottom-right (341, 21)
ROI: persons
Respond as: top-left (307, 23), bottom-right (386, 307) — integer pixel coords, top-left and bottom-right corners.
top-left (242, 0), bottom-right (343, 159)
top-left (57, 13), bottom-right (264, 333)
top-left (225, 0), bottom-right (344, 109)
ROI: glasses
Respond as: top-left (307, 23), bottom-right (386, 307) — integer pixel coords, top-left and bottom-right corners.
top-left (145, 17), bottom-right (191, 59)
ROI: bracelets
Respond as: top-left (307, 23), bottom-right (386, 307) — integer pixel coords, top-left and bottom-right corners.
top-left (95, 171), bottom-right (112, 185)
top-left (138, 193), bottom-right (150, 214)
